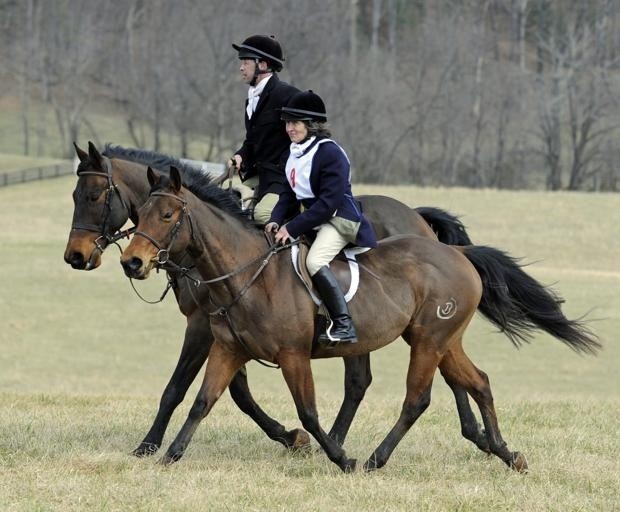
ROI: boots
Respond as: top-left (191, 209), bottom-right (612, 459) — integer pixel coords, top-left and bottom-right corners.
top-left (310, 265), bottom-right (359, 345)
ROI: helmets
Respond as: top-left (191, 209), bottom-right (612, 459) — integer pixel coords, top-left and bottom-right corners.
top-left (280, 89), bottom-right (328, 123)
top-left (232, 34), bottom-right (285, 73)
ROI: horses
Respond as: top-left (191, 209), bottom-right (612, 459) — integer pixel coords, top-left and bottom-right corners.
top-left (120, 164), bottom-right (603, 475)
top-left (63, 140), bottom-right (543, 457)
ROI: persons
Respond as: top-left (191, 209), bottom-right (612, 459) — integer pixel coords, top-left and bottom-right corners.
top-left (231, 33), bottom-right (301, 227)
top-left (264, 89), bottom-right (378, 349)
